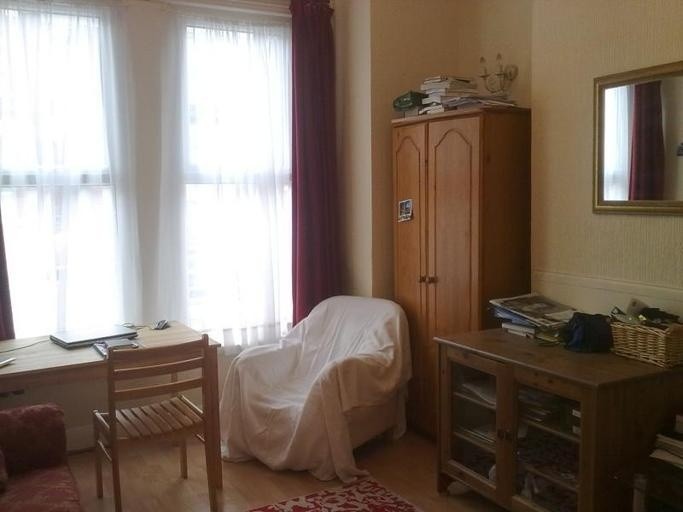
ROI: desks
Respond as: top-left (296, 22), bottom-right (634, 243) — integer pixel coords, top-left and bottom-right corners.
top-left (0, 319), bottom-right (221, 445)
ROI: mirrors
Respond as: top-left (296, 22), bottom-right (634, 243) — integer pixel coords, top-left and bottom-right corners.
top-left (588, 59), bottom-right (683, 216)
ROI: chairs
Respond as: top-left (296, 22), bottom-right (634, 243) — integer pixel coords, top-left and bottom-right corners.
top-left (0, 401), bottom-right (80, 510)
top-left (91, 334), bottom-right (219, 511)
top-left (231, 295), bottom-right (410, 465)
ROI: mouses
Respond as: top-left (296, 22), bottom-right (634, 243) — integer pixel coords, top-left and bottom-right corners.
top-left (155, 320), bottom-right (167, 329)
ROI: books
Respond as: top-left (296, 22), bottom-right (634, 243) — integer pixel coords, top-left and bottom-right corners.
top-left (417, 75), bottom-right (515, 116)
top-left (489, 292), bottom-right (586, 340)
top-left (94, 338), bottom-right (148, 360)
top-left (458, 372), bottom-right (581, 436)
top-left (648, 412), bottom-right (683, 470)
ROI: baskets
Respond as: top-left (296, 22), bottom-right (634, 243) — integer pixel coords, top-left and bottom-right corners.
top-left (610, 322), bottom-right (683, 368)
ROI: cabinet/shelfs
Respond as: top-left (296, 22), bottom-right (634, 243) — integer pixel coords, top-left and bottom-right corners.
top-left (388, 104), bottom-right (528, 440)
top-left (429, 323), bottom-right (681, 511)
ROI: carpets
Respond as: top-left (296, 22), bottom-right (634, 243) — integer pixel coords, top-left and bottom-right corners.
top-left (245, 474), bottom-right (423, 511)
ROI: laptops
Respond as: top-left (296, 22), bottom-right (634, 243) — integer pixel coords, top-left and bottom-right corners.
top-left (50, 322), bottom-right (137, 348)
